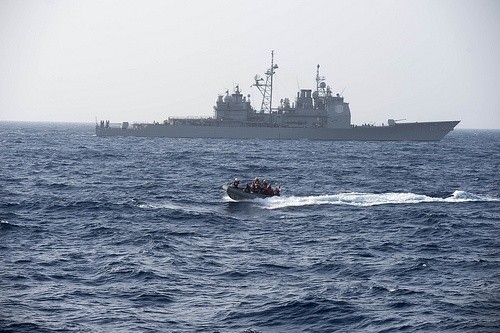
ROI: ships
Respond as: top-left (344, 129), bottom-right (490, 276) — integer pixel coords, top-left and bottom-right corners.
top-left (95, 48), bottom-right (461, 141)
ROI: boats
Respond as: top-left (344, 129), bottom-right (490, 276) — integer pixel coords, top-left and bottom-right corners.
top-left (225, 184), bottom-right (280, 202)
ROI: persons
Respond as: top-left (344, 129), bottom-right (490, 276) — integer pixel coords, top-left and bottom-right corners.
top-left (233, 176), bottom-right (280, 198)
